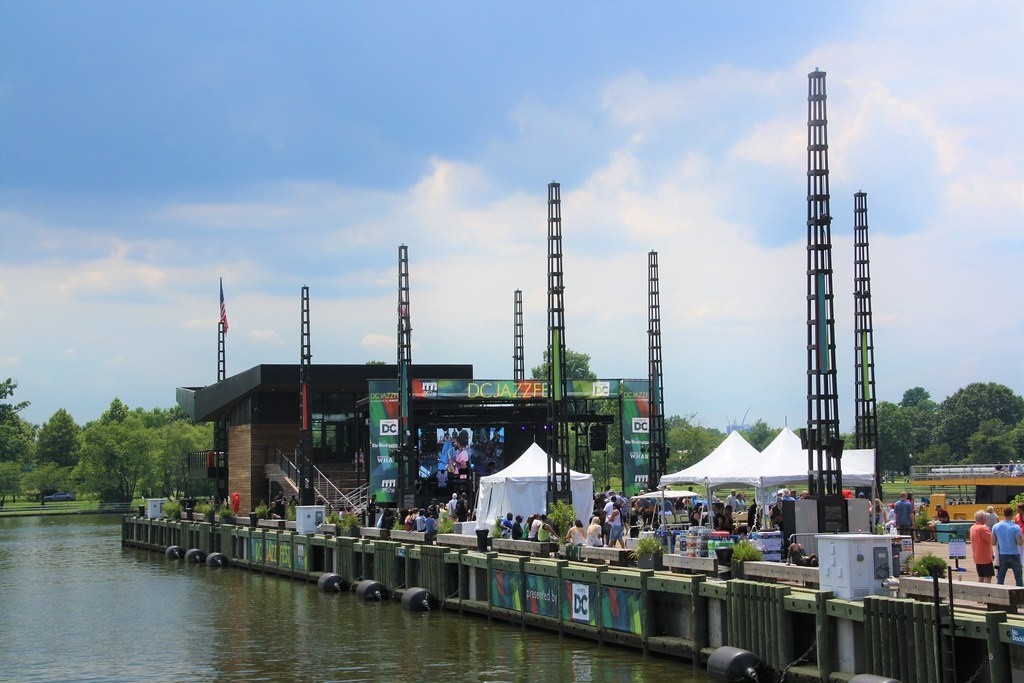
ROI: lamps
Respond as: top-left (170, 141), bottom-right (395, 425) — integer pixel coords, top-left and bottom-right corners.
top-left (640, 443), bottom-right (661, 452)
top-left (583, 422), bottom-right (591, 431)
top-left (571, 423), bottom-right (578, 431)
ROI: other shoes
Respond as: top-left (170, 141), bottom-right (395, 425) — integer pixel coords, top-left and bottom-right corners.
top-left (786, 562), bottom-right (791, 566)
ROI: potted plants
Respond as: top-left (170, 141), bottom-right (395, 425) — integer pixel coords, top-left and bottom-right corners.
top-left (340, 512), bottom-right (360, 536)
top-left (730, 541), bottom-right (762, 581)
top-left (284, 501), bottom-right (296, 530)
top-left (219, 506), bottom-right (234, 524)
top-left (627, 537), bottom-right (667, 569)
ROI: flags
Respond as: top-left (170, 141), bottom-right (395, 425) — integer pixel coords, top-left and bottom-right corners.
top-left (219, 278), bottom-right (228, 337)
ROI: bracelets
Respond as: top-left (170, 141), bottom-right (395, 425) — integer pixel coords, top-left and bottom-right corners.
top-left (453, 461), bottom-right (456, 466)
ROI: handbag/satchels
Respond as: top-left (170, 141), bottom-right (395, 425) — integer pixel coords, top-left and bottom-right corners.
top-left (458, 461), bottom-right (470, 475)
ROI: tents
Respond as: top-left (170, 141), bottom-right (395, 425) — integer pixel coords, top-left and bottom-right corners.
top-left (474, 443), bottom-right (594, 533)
top-left (659, 430), bottom-right (761, 530)
top-left (761, 428), bottom-right (877, 533)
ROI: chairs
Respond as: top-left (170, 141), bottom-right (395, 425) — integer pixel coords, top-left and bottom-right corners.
top-left (658, 509), bottom-right (748, 531)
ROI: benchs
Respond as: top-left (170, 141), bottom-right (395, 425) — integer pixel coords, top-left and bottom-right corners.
top-left (319, 524), bottom-right (339, 535)
top-left (662, 554), bottom-right (731, 579)
top-left (491, 538), bottom-right (557, 558)
top-left (559, 545), bottom-right (634, 567)
top-left (235, 517), bottom-right (250, 525)
top-left (180, 513), bottom-right (219, 523)
top-left (899, 575), bottom-right (1024, 613)
top-left (744, 561), bottom-right (819, 589)
top-left (390, 530), bottom-right (432, 544)
top-left (360, 527), bottom-right (387, 539)
top-left (437, 534), bottom-right (494, 552)
top-left (258, 519), bottom-right (285, 529)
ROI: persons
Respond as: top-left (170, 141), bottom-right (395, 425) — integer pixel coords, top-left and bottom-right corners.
top-left (185, 485), bottom-right (818, 568)
top-left (857, 460), bottom-right (1024, 608)
top-left (353, 446), bottom-right (364, 472)
top-left (447, 430), bottom-right (500, 483)
